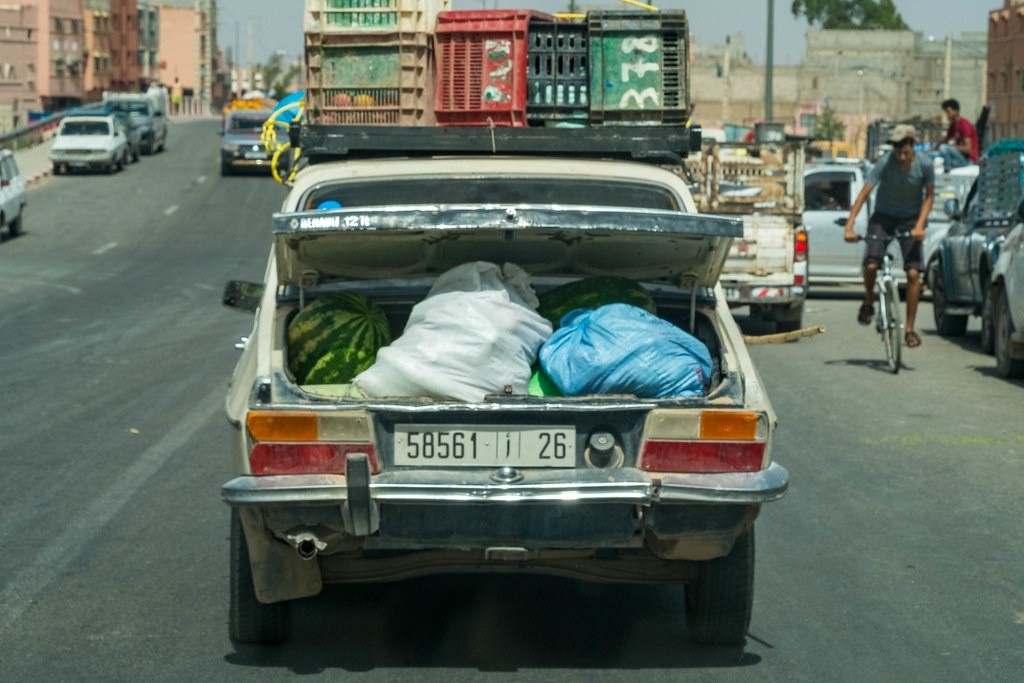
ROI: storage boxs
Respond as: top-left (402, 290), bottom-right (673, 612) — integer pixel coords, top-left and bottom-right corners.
top-left (304, 30), bottom-right (435, 127)
top-left (527, 21), bottom-right (589, 122)
top-left (586, 9), bottom-right (692, 127)
top-left (432, 9), bottom-right (559, 127)
top-left (304, 0), bottom-right (451, 35)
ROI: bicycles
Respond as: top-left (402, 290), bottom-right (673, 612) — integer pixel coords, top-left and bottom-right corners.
top-left (852, 231), bottom-right (912, 375)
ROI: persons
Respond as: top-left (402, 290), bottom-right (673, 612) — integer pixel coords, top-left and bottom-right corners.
top-left (843, 125), bottom-right (935, 348)
top-left (930, 99), bottom-right (978, 173)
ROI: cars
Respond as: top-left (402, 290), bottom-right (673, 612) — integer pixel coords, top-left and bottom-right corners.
top-left (49, 109), bottom-right (129, 174)
top-left (216, 108), bottom-right (294, 176)
top-left (804, 140), bottom-right (1024, 382)
top-left (0, 148), bottom-right (30, 240)
top-left (213, 152), bottom-right (792, 650)
top-left (96, 93), bottom-right (169, 154)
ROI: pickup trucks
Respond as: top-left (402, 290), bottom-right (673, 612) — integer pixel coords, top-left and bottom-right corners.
top-left (677, 147), bottom-right (810, 334)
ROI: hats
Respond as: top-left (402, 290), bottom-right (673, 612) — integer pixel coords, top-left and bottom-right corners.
top-left (885, 124), bottom-right (919, 145)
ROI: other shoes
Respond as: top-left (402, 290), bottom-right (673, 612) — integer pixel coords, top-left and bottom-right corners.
top-left (858, 304), bottom-right (875, 324)
top-left (905, 331), bottom-right (921, 348)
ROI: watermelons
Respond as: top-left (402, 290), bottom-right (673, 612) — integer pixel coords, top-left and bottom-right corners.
top-left (289, 291), bottom-right (391, 387)
top-left (537, 276), bottom-right (656, 330)
